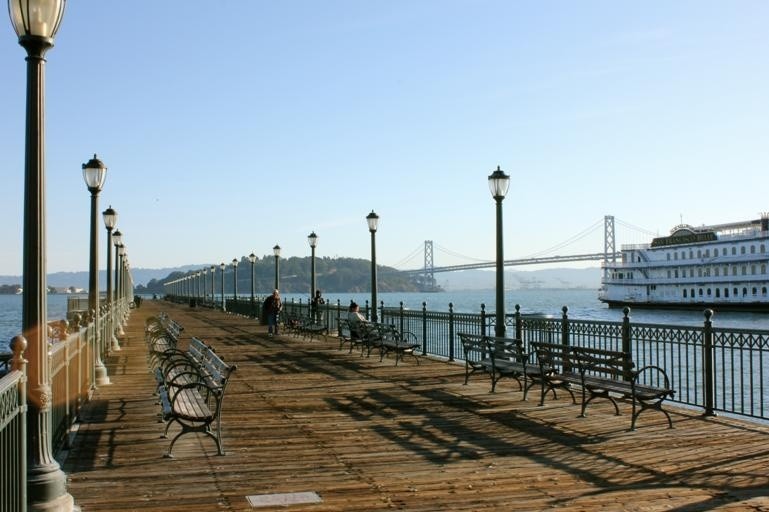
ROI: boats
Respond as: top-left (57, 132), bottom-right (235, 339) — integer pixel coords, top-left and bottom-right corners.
top-left (598, 213), bottom-right (769, 309)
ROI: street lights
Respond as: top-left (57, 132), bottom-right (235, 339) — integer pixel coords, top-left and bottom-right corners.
top-left (487, 165), bottom-right (511, 363)
top-left (366, 210), bottom-right (380, 323)
top-left (6, 1), bottom-right (76, 512)
top-left (164, 244), bottom-right (282, 311)
top-left (307, 231), bottom-right (319, 324)
top-left (82, 154), bottom-right (134, 386)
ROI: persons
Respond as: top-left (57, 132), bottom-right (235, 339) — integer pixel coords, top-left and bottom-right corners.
top-left (263, 289), bottom-right (283, 338)
top-left (310, 290), bottom-right (325, 326)
top-left (348, 300), bottom-right (380, 336)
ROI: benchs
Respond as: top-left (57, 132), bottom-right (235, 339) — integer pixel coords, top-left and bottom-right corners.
top-left (335, 316), bottom-right (422, 367)
top-left (281, 311), bottom-right (329, 342)
top-left (149, 319), bottom-right (237, 459)
top-left (455, 328), bottom-right (678, 433)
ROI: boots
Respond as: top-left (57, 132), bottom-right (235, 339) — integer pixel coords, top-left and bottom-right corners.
top-left (268, 326), bottom-right (273, 337)
top-left (275, 326), bottom-right (281, 335)
